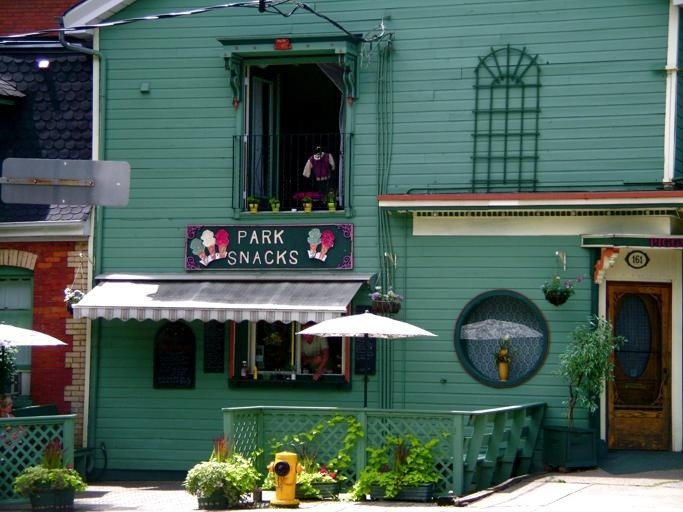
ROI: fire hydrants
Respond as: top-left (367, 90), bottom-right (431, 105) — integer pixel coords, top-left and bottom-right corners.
top-left (267, 451), bottom-right (304, 509)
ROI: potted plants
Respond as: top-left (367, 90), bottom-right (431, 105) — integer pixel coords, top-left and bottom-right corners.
top-left (302, 196), bottom-right (313, 213)
top-left (247, 196), bottom-right (260, 213)
top-left (544, 312), bottom-right (629, 471)
top-left (327, 193), bottom-right (338, 211)
top-left (351, 429), bottom-right (451, 502)
top-left (12, 440), bottom-right (87, 511)
top-left (269, 197), bottom-right (281, 213)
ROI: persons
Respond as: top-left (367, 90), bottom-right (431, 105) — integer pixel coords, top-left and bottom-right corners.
top-left (300, 321), bottom-right (330, 383)
top-left (0, 392), bottom-right (32, 500)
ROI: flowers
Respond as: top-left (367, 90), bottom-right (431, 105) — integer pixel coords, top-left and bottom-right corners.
top-left (293, 461), bottom-right (345, 495)
top-left (544, 275), bottom-right (582, 296)
top-left (181, 433), bottom-right (258, 505)
top-left (369, 286), bottom-right (404, 304)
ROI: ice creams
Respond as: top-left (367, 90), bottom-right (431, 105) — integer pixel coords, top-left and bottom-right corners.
top-left (319, 230), bottom-right (335, 259)
top-left (190, 229), bottom-right (229, 261)
top-left (307, 228), bottom-right (321, 252)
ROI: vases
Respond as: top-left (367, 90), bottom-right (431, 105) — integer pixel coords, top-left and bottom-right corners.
top-left (374, 300), bottom-right (401, 314)
top-left (197, 488), bottom-right (230, 510)
top-left (294, 483), bottom-right (341, 501)
top-left (542, 288), bottom-right (572, 306)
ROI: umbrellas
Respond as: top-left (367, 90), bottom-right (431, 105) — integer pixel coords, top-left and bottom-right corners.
top-left (0, 320), bottom-right (69, 348)
top-left (294, 308), bottom-right (438, 408)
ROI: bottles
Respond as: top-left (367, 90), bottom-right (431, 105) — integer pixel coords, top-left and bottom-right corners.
top-left (241, 360), bottom-right (248, 378)
top-left (253, 366), bottom-right (258, 379)
top-left (274, 368), bottom-right (297, 381)
